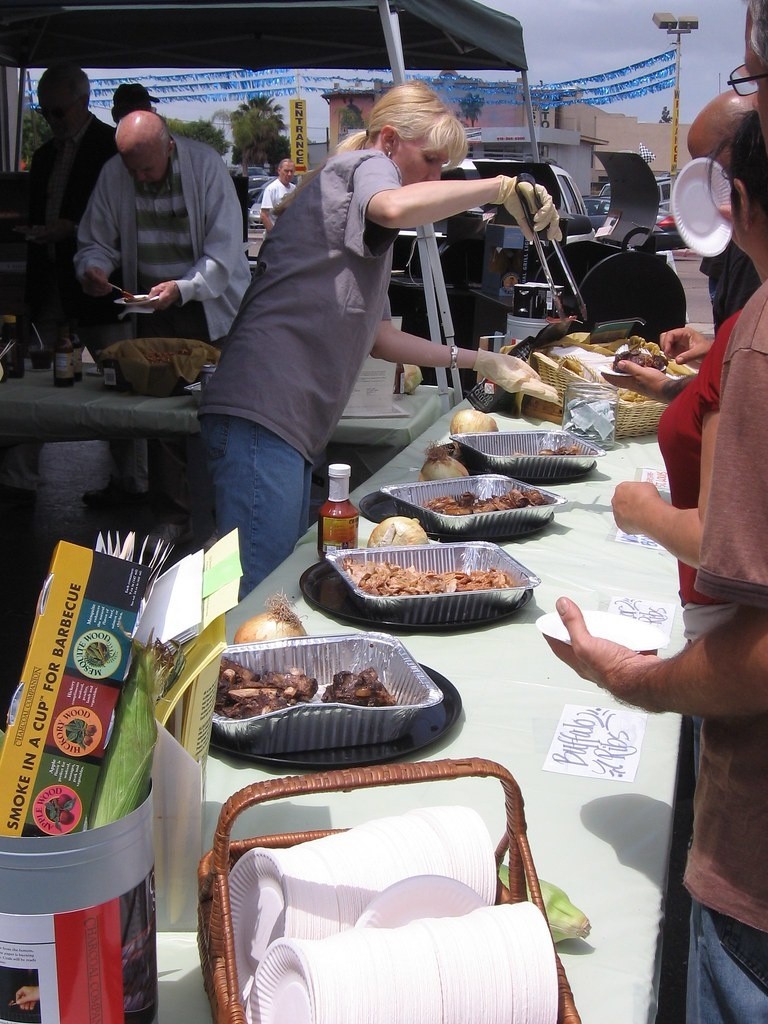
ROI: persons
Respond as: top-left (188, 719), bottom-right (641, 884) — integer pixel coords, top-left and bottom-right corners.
top-left (197, 81), bottom-right (564, 606)
top-left (112, 84), bottom-right (160, 128)
top-left (74, 111), bottom-right (253, 352)
top-left (260, 159), bottom-right (298, 241)
top-left (22, 62), bottom-right (119, 237)
top-left (541, 0), bottom-right (768, 1024)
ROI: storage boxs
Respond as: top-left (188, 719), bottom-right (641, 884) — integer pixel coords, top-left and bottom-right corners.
top-left (0, 540), bottom-right (151, 837)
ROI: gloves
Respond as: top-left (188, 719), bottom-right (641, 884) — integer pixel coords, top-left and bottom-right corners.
top-left (473, 348), bottom-right (559, 403)
top-left (490, 175), bottom-right (563, 242)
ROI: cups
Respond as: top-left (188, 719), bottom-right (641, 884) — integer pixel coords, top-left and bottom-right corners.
top-left (27, 343), bottom-right (55, 369)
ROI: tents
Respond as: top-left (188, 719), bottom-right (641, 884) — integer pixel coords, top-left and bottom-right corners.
top-left (0, 0), bottom-right (546, 413)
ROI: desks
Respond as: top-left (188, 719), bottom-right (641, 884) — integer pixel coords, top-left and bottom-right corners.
top-left (133, 396), bottom-right (690, 1024)
top-left (1, 356), bottom-right (456, 515)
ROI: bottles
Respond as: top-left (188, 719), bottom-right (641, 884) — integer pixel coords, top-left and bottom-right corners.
top-left (0, 315), bottom-right (25, 378)
top-left (317, 463), bottom-right (360, 559)
top-left (69, 318), bottom-right (82, 382)
top-left (53, 325), bottom-right (74, 388)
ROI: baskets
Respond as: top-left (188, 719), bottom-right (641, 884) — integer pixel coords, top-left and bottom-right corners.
top-left (532, 337), bottom-right (669, 440)
top-left (196, 758), bottom-right (584, 1024)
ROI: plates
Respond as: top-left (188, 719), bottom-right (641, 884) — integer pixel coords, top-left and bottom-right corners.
top-left (114, 294), bottom-right (159, 305)
top-left (597, 364), bottom-right (666, 377)
top-left (671, 157), bottom-right (735, 257)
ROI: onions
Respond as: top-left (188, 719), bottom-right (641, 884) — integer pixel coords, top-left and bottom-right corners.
top-left (418, 442), bottom-right (471, 482)
top-left (234, 590), bottom-right (306, 645)
top-left (450, 409), bottom-right (499, 433)
top-left (403, 364), bottom-right (423, 392)
top-left (366, 516), bottom-right (430, 547)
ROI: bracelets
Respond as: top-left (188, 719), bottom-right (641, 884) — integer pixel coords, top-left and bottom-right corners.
top-left (450, 343), bottom-right (459, 370)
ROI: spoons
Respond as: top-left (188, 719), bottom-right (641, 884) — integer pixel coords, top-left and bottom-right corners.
top-left (106, 283), bottom-right (134, 299)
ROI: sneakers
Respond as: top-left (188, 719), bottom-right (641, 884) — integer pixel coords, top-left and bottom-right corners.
top-left (82, 482), bottom-right (148, 510)
top-left (0, 484), bottom-right (36, 508)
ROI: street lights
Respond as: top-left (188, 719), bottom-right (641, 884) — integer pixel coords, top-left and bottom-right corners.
top-left (653, 12), bottom-right (699, 173)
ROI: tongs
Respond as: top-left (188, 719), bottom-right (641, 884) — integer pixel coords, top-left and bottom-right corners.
top-left (514, 172), bottom-right (588, 322)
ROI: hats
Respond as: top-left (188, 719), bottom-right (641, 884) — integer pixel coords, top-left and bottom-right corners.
top-left (113, 84), bottom-right (160, 105)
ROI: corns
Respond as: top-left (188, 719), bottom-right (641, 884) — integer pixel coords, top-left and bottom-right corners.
top-left (88, 630), bottom-right (186, 829)
top-left (499, 864), bottom-right (591, 943)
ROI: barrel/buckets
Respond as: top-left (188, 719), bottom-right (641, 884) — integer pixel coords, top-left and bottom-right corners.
top-left (504, 312), bottom-right (549, 348)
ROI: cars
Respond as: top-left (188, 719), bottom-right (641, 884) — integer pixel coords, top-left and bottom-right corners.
top-left (232, 164), bottom-right (687, 250)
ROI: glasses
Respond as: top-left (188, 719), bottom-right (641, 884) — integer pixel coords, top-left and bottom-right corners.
top-left (727, 64), bottom-right (768, 96)
top-left (35, 95), bottom-right (84, 118)
